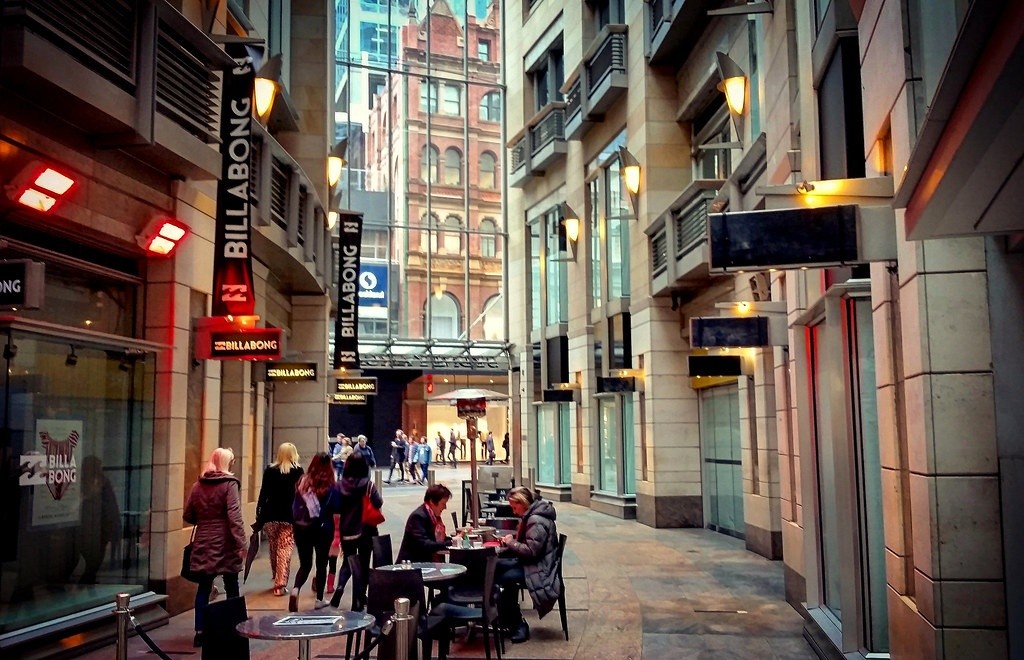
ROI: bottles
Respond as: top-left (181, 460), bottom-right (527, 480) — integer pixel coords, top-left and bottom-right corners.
top-left (401, 559), bottom-right (412, 570)
top-left (499, 490), bottom-right (506, 502)
top-left (488, 513), bottom-right (495, 519)
top-left (462, 532), bottom-right (471, 549)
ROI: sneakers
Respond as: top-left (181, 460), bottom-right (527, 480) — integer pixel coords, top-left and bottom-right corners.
top-left (315, 597), bottom-right (330, 609)
top-left (289, 587), bottom-right (300, 612)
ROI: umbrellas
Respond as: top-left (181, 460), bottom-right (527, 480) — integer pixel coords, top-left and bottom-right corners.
top-left (243, 521), bottom-right (263, 585)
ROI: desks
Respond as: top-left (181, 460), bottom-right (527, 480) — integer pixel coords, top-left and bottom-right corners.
top-left (478, 491), bottom-right (505, 501)
top-left (375, 561), bottom-right (467, 604)
top-left (484, 501), bottom-right (513, 516)
top-left (236, 609), bottom-right (376, 660)
top-left (444, 529), bottom-right (518, 590)
top-left (486, 517), bottom-right (519, 530)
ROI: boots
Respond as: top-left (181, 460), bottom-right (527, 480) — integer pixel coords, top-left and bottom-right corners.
top-left (327, 574), bottom-right (335, 593)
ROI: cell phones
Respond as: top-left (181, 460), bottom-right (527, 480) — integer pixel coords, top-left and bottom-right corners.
top-left (492, 534), bottom-right (502, 540)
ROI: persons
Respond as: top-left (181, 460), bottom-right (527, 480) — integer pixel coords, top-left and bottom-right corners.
top-left (182, 447), bottom-right (249, 649)
top-left (495, 486), bottom-right (562, 644)
top-left (354, 435), bottom-right (377, 479)
top-left (332, 437), bottom-right (354, 464)
top-left (485, 431), bottom-right (494, 466)
top-left (437, 432), bottom-right (447, 465)
top-left (478, 431), bottom-right (487, 459)
top-left (384, 429), bottom-right (432, 486)
top-left (289, 451), bottom-right (340, 611)
top-left (79, 453), bottom-right (121, 584)
top-left (502, 433), bottom-right (510, 462)
top-left (255, 442), bottom-right (304, 595)
top-left (395, 484), bottom-right (466, 591)
top-left (328, 435), bottom-right (331, 456)
top-left (447, 429), bottom-right (456, 468)
top-left (459, 432), bottom-right (467, 460)
top-left (333, 433), bottom-right (345, 481)
top-left (435, 432), bottom-right (443, 462)
top-left (325, 451), bottom-right (384, 613)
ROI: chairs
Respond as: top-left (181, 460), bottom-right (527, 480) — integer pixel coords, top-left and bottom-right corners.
top-left (201, 595), bottom-right (250, 660)
top-left (345, 487), bottom-right (568, 660)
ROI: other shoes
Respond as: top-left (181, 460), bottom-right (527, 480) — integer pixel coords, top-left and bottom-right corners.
top-left (398, 479), bottom-right (424, 485)
top-left (383, 480), bottom-right (390, 484)
top-left (511, 625), bottom-right (530, 643)
top-left (194, 632), bottom-right (203, 647)
top-left (273, 587), bottom-right (287, 596)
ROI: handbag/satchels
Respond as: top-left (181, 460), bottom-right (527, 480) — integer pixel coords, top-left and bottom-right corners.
top-left (181, 523), bottom-right (200, 583)
top-left (489, 452), bottom-right (496, 458)
top-left (361, 480), bottom-right (385, 526)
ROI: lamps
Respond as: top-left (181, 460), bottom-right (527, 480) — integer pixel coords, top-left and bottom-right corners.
top-left (796, 180), bottom-right (816, 193)
top-left (4, 159), bottom-right (78, 215)
top-left (65, 343), bottom-right (79, 367)
top-left (254, 53), bottom-right (283, 118)
top-left (603, 145), bottom-right (642, 220)
top-left (359, 354), bottom-right (498, 368)
top-left (325, 187), bottom-right (342, 230)
top-left (3, 333), bottom-right (17, 360)
top-left (117, 354), bottom-right (131, 372)
top-left (135, 215), bottom-right (190, 258)
top-left (697, 51), bottom-right (748, 151)
top-left (550, 202), bottom-right (579, 262)
top-left (328, 136), bottom-right (349, 187)
